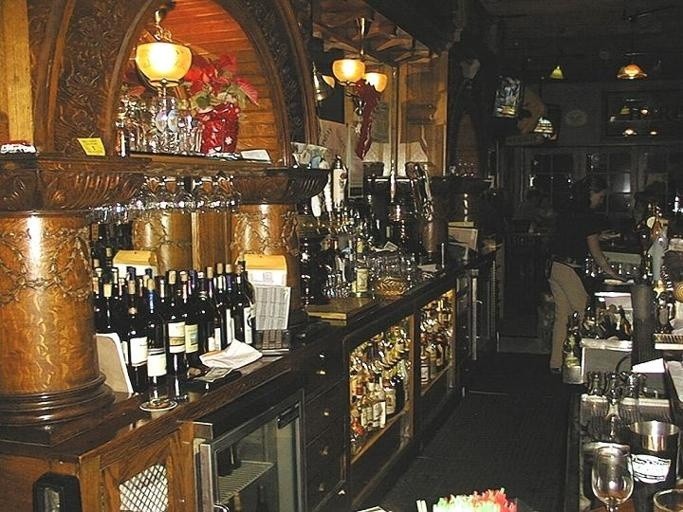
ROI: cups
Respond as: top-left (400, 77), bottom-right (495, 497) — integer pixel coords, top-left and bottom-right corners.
top-left (628, 421), bottom-right (683, 512)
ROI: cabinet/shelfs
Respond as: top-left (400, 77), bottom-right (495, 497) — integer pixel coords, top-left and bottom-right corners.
top-left (0, 0), bottom-right (509, 512)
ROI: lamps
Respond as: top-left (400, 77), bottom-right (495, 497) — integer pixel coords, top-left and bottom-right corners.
top-left (547, 61), bottom-right (563, 80)
top-left (614, 9), bottom-right (648, 82)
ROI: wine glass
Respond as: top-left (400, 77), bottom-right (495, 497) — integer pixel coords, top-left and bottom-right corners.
top-left (590, 445), bottom-right (634, 512)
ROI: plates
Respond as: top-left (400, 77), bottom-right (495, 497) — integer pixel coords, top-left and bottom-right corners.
top-left (139, 401), bottom-right (178, 412)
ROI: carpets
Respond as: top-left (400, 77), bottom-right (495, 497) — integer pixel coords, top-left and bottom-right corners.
top-left (375, 350), bottom-right (573, 512)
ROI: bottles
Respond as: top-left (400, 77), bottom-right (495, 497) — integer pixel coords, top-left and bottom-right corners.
top-left (92, 247), bottom-right (256, 391)
top-left (562, 301), bottom-right (653, 446)
top-left (348, 314), bottom-right (409, 456)
top-left (202, 156), bottom-right (203, 159)
top-left (421, 290), bottom-right (454, 389)
top-left (350, 234), bottom-right (372, 298)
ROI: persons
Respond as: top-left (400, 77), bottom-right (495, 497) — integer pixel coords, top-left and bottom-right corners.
top-left (544, 174), bottom-right (626, 375)
top-left (479, 180), bottom-right (683, 263)
